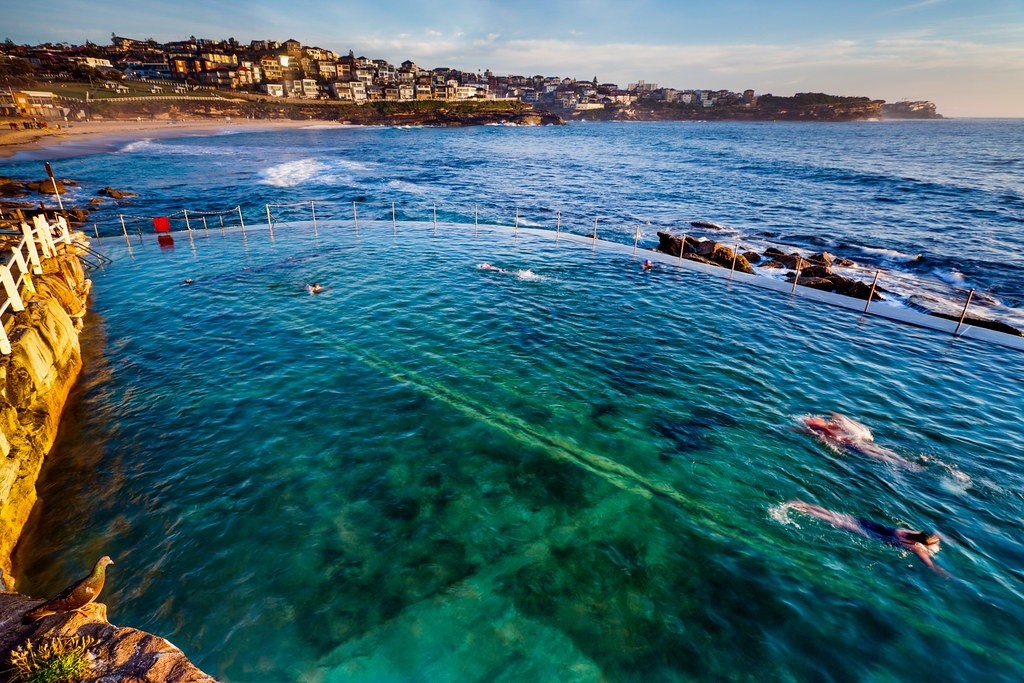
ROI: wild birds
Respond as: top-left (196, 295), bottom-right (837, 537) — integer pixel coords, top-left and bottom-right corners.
top-left (18, 555), bottom-right (114, 622)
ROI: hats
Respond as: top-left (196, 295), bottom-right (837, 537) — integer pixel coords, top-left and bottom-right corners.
top-left (920, 528), bottom-right (939, 545)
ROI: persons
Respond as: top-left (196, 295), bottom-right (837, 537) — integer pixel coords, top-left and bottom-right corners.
top-left (306, 282), bottom-right (328, 296)
top-left (9, 121), bottom-right (48, 130)
top-left (483, 263), bottom-right (506, 271)
top-left (805, 412), bottom-right (929, 472)
top-left (790, 497), bottom-right (953, 581)
